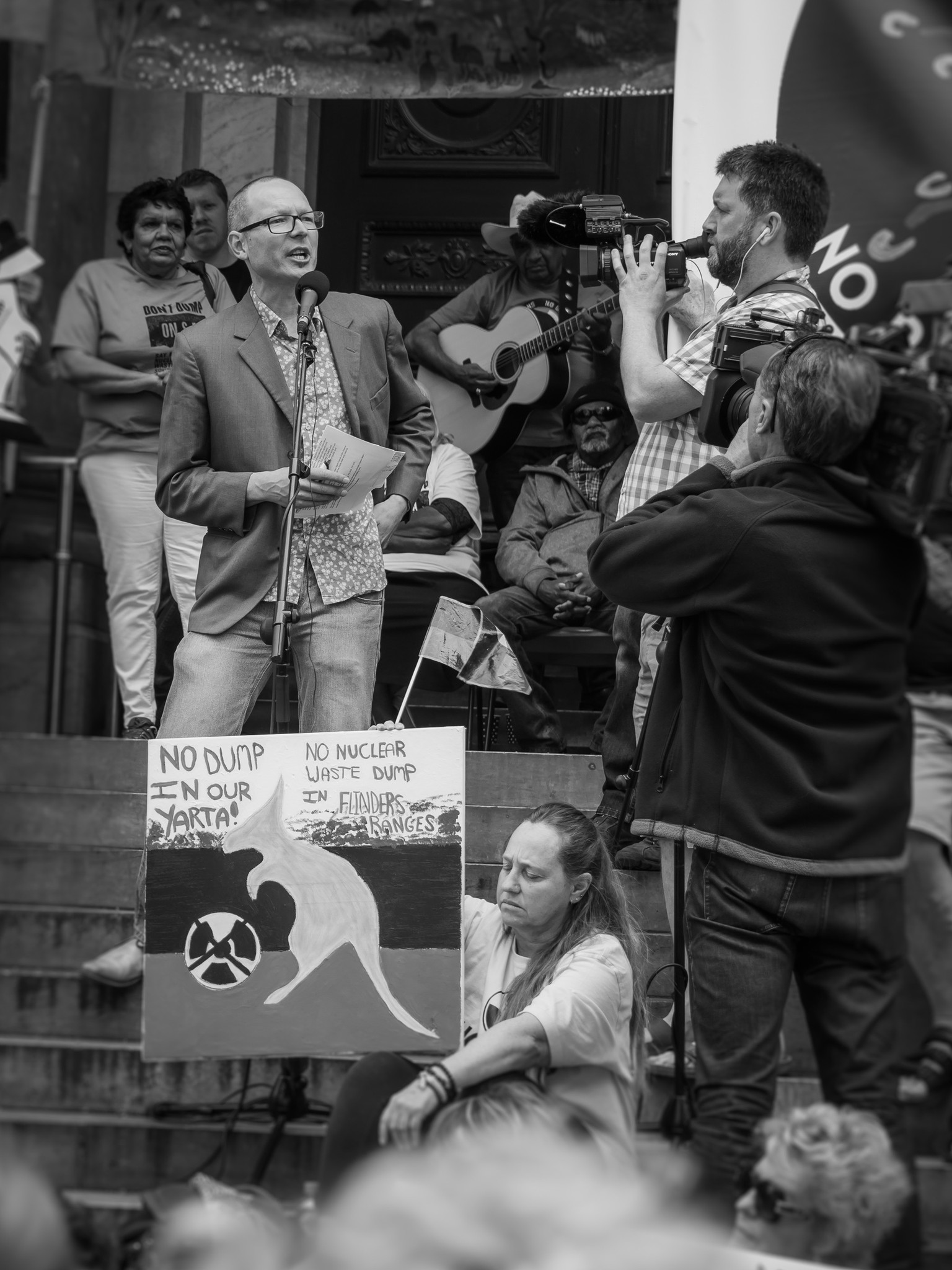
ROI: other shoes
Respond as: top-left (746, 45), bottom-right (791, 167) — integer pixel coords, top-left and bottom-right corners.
top-left (587, 814), bottom-right (641, 854)
top-left (614, 833), bottom-right (665, 869)
top-left (124, 715), bottom-right (156, 741)
top-left (82, 940), bottom-right (144, 989)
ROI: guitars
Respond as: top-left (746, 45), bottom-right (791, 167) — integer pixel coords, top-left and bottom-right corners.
top-left (417, 291), bottom-right (621, 464)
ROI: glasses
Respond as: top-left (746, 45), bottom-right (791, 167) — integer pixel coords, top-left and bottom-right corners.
top-left (770, 333), bottom-right (849, 434)
top-left (574, 406), bottom-right (623, 426)
top-left (239, 212), bottom-right (325, 234)
top-left (741, 1170), bottom-right (816, 1224)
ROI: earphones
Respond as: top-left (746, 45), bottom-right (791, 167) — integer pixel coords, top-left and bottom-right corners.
top-left (756, 226), bottom-right (771, 243)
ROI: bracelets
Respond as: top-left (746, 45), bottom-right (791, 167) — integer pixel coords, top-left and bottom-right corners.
top-left (418, 1062), bottom-right (457, 1108)
top-left (592, 339), bottom-right (614, 355)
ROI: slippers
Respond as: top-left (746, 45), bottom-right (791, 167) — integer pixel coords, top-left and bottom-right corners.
top-left (642, 1016), bottom-right (791, 1078)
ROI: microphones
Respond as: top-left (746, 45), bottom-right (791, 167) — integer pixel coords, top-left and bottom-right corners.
top-left (295, 271), bottom-right (330, 335)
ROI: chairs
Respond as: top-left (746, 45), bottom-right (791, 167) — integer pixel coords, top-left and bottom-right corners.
top-left (384, 573), bottom-right (618, 751)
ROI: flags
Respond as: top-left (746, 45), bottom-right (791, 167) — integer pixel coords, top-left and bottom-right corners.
top-left (420, 597), bottom-right (532, 695)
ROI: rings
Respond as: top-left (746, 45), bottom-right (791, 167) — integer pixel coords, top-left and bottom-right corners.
top-left (476, 388), bottom-right (481, 395)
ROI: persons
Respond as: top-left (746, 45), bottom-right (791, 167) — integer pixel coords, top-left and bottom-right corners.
top-left (611, 140), bottom-right (842, 1080)
top-left (50, 178), bottom-right (236, 739)
top-left (0, 218), bottom-right (44, 363)
top-left (81, 178), bottom-right (435, 982)
top-left (588, 331), bottom-right (932, 1270)
top-left (901, 449), bottom-right (952, 1098)
top-left (733, 1104), bottom-right (911, 1270)
top-left (155, 167), bottom-right (252, 730)
top-left (373, 383), bottom-right (491, 722)
top-left (311, 720), bottom-right (650, 1216)
top-left (473, 379), bottom-right (661, 869)
top-left (405, 191), bottom-right (623, 532)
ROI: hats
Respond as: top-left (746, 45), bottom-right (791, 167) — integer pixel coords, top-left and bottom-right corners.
top-left (562, 379), bottom-right (634, 429)
top-left (480, 191), bottom-right (549, 255)
